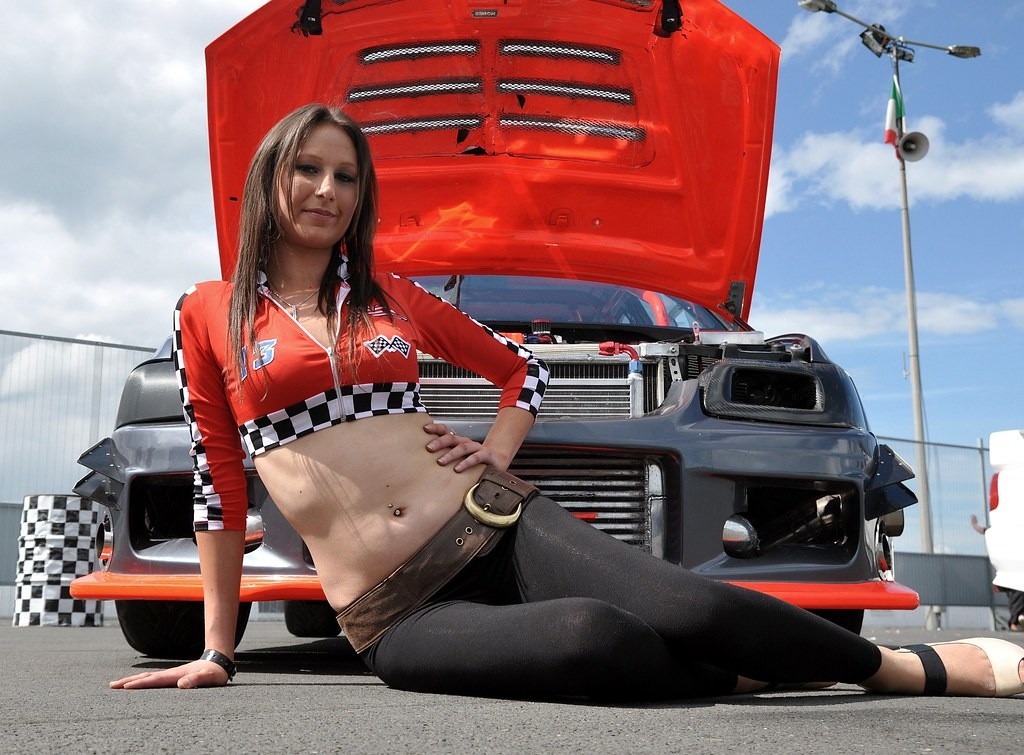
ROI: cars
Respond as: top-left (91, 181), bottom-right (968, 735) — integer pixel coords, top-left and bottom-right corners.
top-left (70, 0), bottom-right (918, 660)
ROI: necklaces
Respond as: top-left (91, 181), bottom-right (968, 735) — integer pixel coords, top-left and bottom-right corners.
top-left (269, 288), bottom-right (319, 323)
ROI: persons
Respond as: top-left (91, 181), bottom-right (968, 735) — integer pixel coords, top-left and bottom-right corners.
top-left (112, 103), bottom-right (1024, 705)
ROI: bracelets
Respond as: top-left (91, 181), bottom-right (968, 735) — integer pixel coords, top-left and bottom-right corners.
top-left (200, 649), bottom-right (237, 682)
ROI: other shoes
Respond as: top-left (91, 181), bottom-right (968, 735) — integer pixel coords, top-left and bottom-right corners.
top-left (896, 639), bottom-right (1024, 699)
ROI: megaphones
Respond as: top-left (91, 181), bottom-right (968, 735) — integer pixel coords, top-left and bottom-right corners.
top-left (895, 131), bottom-right (930, 162)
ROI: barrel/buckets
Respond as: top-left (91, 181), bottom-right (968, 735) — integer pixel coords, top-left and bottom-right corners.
top-left (9, 493), bottom-right (109, 628)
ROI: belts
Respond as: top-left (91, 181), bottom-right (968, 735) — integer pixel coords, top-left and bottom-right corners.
top-left (333, 468), bottom-right (541, 654)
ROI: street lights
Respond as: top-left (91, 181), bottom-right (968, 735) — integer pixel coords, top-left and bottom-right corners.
top-left (798, 0), bottom-right (981, 631)
